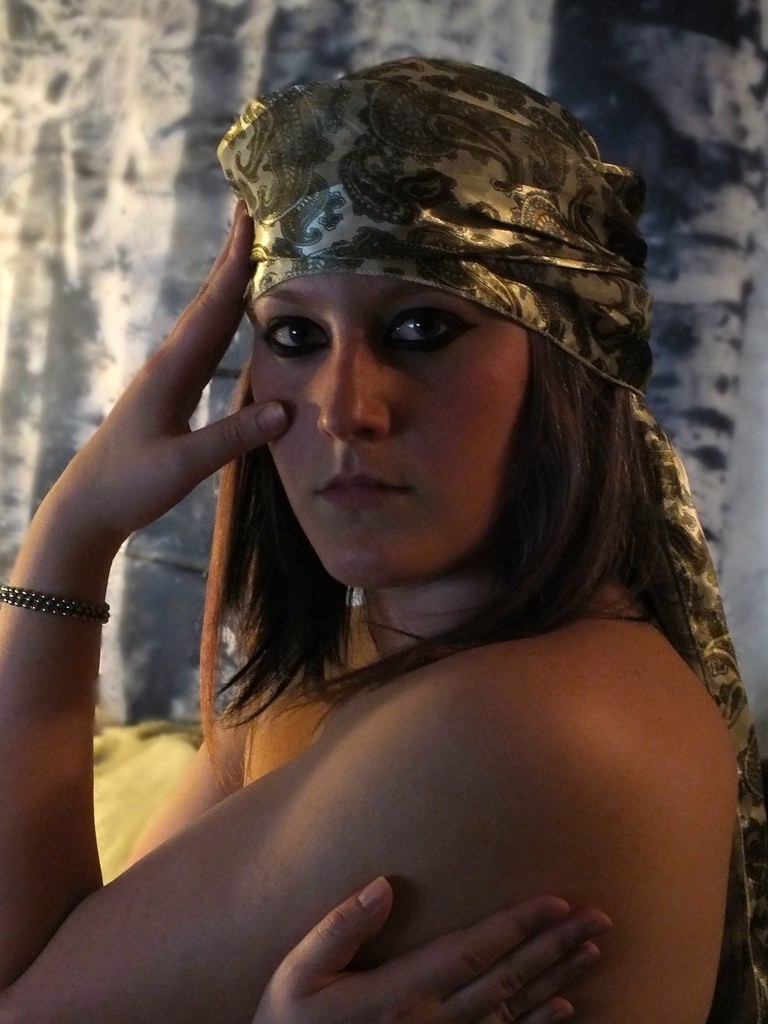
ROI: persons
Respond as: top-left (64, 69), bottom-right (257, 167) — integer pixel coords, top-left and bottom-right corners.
top-left (1, 56), bottom-right (767, 1024)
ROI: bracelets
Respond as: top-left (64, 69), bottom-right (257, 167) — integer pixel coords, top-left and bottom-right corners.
top-left (1, 584), bottom-right (110, 626)
